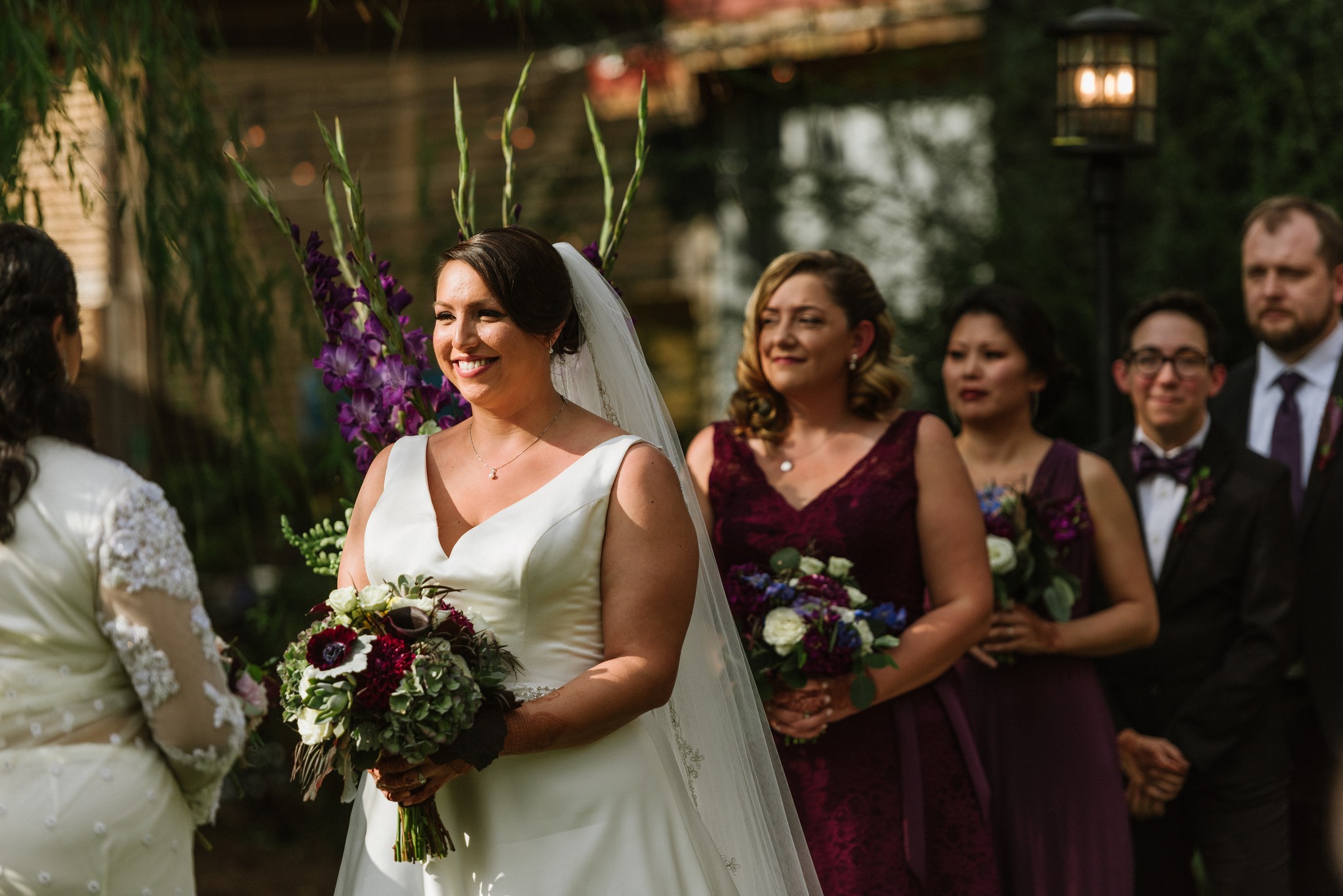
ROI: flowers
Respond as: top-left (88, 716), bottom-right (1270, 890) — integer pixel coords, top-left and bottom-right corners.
top-left (970, 475), bottom-right (1077, 637)
top-left (267, 571), bottom-right (527, 862)
top-left (719, 544), bottom-right (907, 746)
top-left (208, 56), bottom-right (647, 578)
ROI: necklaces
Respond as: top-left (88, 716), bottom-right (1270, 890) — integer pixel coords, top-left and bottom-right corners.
top-left (780, 408), bottom-right (844, 475)
top-left (468, 392), bottom-right (577, 480)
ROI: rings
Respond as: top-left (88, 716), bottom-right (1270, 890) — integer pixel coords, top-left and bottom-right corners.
top-left (419, 771), bottom-right (428, 785)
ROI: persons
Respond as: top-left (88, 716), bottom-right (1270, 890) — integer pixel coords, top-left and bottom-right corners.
top-left (1205, 193), bottom-right (1343, 896)
top-left (334, 226), bottom-right (821, 896)
top-left (684, 247), bottom-right (997, 896)
top-left (922, 283), bottom-right (1161, 895)
top-left (1089, 294), bottom-right (1299, 896)
top-left (0, 217), bottom-right (255, 896)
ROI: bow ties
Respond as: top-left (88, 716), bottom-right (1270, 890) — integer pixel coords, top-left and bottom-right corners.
top-left (1124, 443), bottom-right (1198, 490)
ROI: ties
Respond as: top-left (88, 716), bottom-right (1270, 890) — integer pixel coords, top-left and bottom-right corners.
top-left (1265, 370), bottom-right (1307, 539)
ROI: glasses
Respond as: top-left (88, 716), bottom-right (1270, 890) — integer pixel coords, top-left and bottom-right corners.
top-left (1121, 344), bottom-right (1219, 383)
